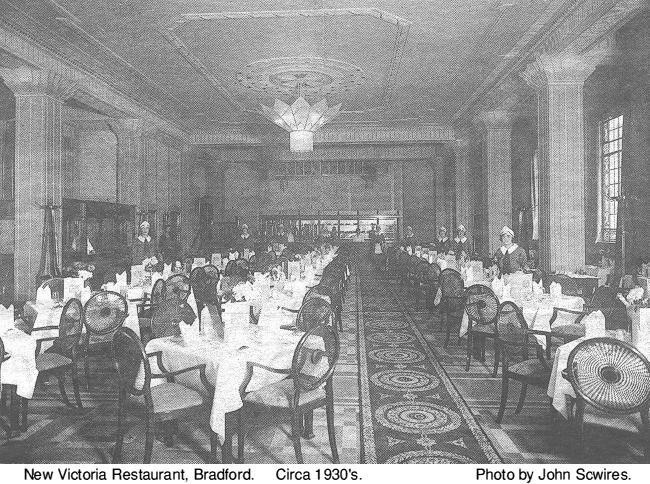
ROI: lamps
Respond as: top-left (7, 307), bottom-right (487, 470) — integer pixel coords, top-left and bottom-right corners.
top-left (259, 83), bottom-right (344, 153)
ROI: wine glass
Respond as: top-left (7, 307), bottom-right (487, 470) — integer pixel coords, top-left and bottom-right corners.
top-left (401, 246), bottom-right (573, 305)
top-left (50, 245), bottom-right (359, 354)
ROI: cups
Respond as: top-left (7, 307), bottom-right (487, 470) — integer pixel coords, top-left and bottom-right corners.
top-left (616, 328), bottom-right (627, 341)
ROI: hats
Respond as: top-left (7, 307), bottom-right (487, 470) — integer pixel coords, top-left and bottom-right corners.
top-left (499, 225), bottom-right (515, 239)
top-left (139, 221), bottom-right (151, 229)
top-left (242, 223), bottom-right (250, 230)
top-left (439, 225), bottom-right (447, 232)
top-left (456, 224), bottom-right (467, 233)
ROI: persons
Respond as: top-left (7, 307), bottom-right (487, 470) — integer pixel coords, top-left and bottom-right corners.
top-left (134, 220), bottom-right (529, 278)
